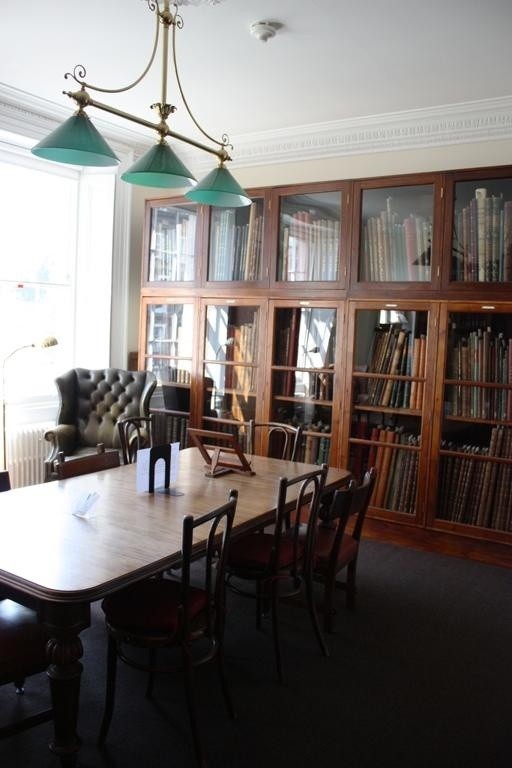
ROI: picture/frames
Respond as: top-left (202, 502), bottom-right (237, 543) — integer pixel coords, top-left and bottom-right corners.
top-left (186, 425), bottom-right (254, 478)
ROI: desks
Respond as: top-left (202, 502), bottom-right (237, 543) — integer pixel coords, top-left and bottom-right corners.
top-left (0, 446), bottom-right (351, 768)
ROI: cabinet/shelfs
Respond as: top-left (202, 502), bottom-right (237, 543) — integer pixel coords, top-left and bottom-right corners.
top-left (139, 165), bottom-right (512, 568)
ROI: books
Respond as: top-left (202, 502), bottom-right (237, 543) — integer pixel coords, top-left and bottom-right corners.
top-left (353, 322), bottom-right (427, 411)
top-left (358, 197), bottom-right (431, 280)
top-left (208, 202), bottom-right (259, 281)
top-left (203, 410), bottom-right (254, 455)
top-left (452, 185), bottom-right (512, 281)
top-left (437, 427), bottom-right (507, 527)
top-left (276, 207), bottom-right (340, 281)
top-left (270, 410), bottom-right (330, 465)
top-left (152, 215), bottom-right (196, 280)
top-left (444, 325), bottom-right (512, 421)
top-left (230, 314), bottom-right (260, 391)
top-left (346, 421), bottom-right (415, 512)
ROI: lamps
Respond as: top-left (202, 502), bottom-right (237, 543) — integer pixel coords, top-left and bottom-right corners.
top-left (0, 337), bottom-right (58, 492)
top-left (122, 142), bottom-right (199, 189)
top-left (29, 110), bottom-right (120, 166)
top-left (185, 164), bottom-right (252, 208)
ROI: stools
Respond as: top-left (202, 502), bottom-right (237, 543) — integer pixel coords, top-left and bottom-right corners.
top-left (0, 599), bottom-right (52, 694)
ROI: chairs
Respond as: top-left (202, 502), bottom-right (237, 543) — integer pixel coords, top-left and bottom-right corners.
top-left (97, 488), bottom-right (238, 762)
top-left (55, 442), bottom-right (120, 480)
top-left (249, 418), bottom-right (303, 462)
top-left (43, 369), bottom-right (157, 481)
top-left (216, 463), bottom-right (329, 678)
top-left (281, 467), bottom-right (377, 634)
